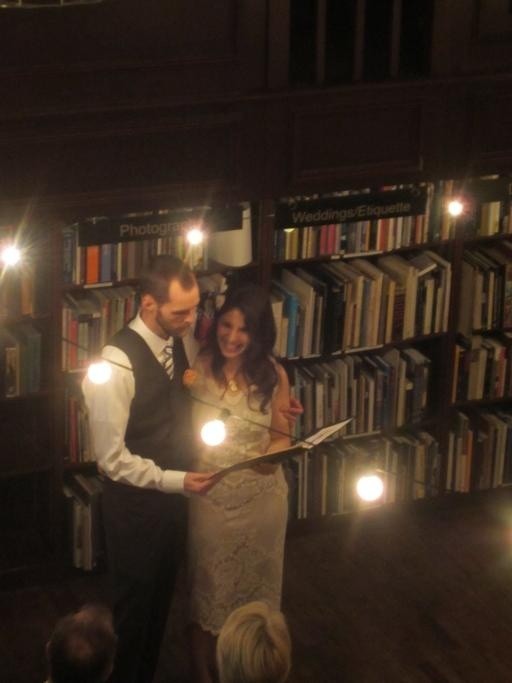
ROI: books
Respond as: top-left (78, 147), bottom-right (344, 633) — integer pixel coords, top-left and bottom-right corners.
top-left (1, 253), bottom-right (41, 402)
top-left (62, 170), bottom-right (512, 570)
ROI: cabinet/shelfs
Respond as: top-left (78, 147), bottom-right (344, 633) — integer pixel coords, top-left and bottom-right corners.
top-left (44, 146), bottom-right (511, 594)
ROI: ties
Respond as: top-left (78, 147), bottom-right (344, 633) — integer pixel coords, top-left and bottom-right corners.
top-left (164, 346), bottom-right (175, 379)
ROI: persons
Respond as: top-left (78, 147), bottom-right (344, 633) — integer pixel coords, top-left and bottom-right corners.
top-left (43, 601), bottom-right (116, 679)
top-left (215, 600), bottom-right (288, 682)
top-left (184, 280), bottom-right (295, 681)
top-left (87, 253), bottom-right (306, 682)
